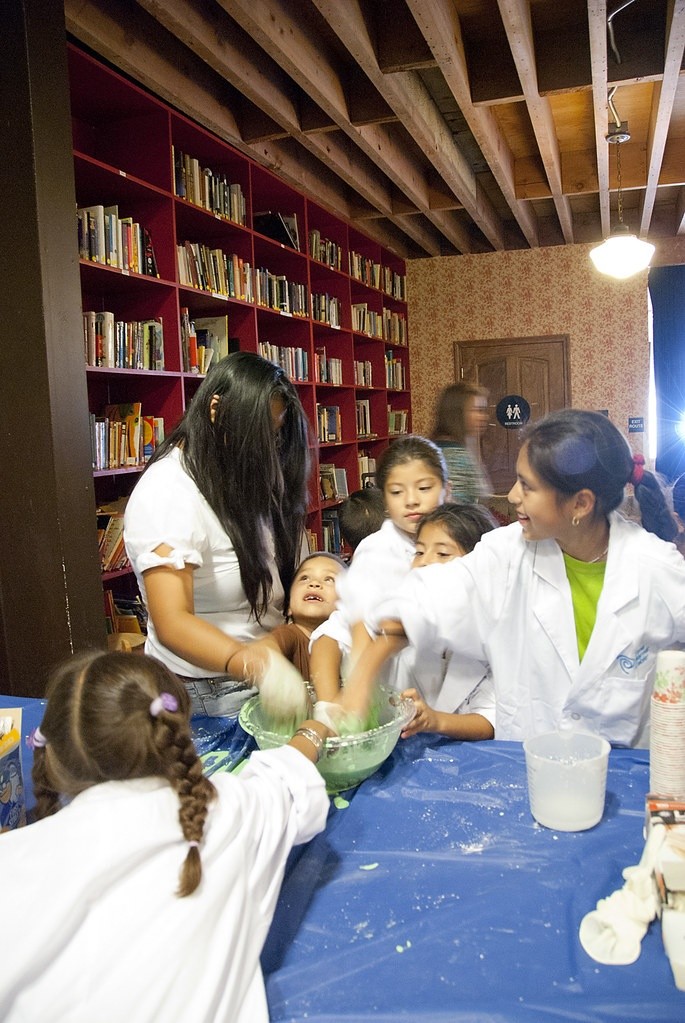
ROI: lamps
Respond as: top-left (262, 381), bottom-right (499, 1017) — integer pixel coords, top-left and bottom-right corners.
top-left (588, 123), bottom-right (655, 278)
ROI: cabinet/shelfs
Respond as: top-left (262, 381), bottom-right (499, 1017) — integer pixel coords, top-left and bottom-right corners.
top-left (65, 39), bottom-right (408, 653)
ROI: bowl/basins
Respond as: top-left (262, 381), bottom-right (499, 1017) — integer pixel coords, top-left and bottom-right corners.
top-left (238, 681), bottom-right (417, 790)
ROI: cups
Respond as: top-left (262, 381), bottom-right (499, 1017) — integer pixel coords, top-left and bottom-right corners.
top-left (649, 650), bottom-right (685, 801)
top-left (523, 729), bottom-right (611, 831)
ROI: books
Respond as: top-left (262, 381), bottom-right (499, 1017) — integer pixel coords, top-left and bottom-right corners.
top-left (77, 145), bottom-right (408, 636)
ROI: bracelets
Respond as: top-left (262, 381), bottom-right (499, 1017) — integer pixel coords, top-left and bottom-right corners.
top-left (225, 648), bottom-right (250, 673)
top-left (292, 727), bottom-right (323, 762)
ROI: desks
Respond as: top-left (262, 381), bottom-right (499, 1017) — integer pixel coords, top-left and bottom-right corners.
top-left (0, 691), bottom-right (685, 1022)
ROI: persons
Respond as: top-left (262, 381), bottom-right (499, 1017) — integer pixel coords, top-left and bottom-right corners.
top-left (1, 651), bottom-right (340, 1023)
top-left (258, 382), bottom-right (684, 748)
top-left (125, 354), bottom-right (310, 779)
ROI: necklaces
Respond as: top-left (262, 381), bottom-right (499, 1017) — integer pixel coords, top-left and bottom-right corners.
top-left (590, 548), bottom-right (608, 562)
